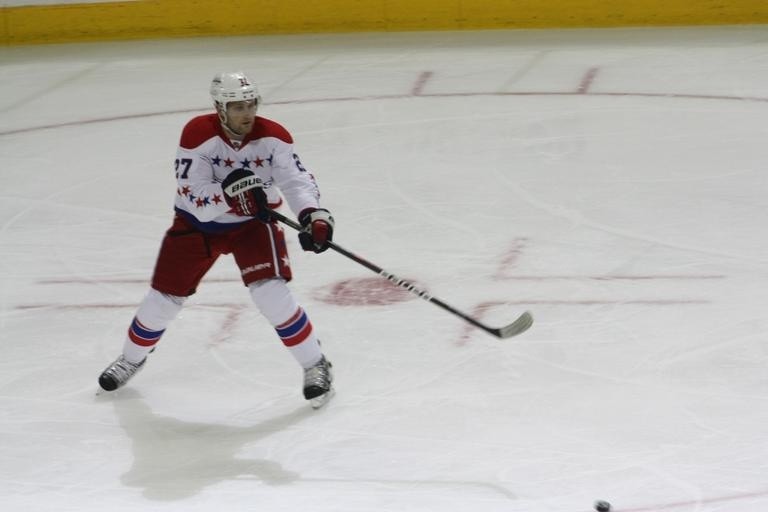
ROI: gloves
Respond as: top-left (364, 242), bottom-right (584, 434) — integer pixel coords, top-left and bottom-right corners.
top-left (221, 168), bottom-right (267, 216)
top-left (298, 208), bottom-right (335, 254)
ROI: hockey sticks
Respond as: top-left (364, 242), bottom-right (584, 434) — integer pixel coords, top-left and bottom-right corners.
top-left (263, 209), bottom-right (533, 339)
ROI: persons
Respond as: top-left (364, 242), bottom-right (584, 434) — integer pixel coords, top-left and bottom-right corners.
top-left (96, 68), bottom-right (335, 402)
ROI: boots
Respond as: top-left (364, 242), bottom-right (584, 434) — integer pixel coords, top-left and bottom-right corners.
top-left (98, 347), bottom-right (155, 392)
top-left (303, 353), bottom-right (331, 400)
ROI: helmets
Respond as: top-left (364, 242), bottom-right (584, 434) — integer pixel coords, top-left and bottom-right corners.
top-left (209, 72), bottom-right (261, 124)
